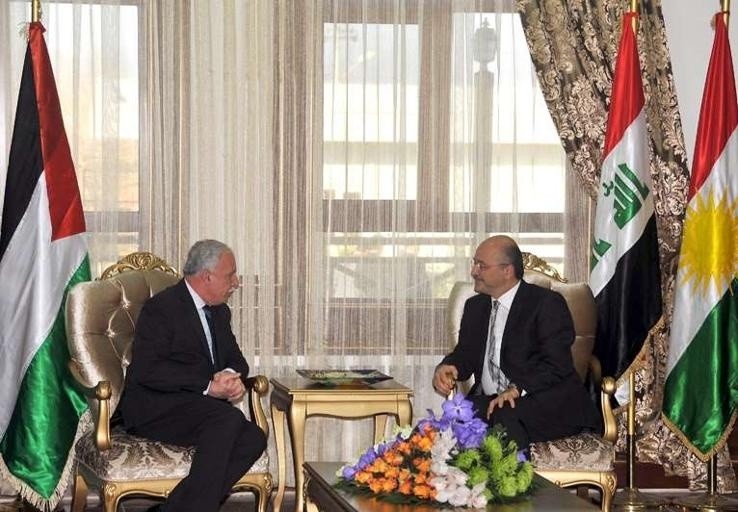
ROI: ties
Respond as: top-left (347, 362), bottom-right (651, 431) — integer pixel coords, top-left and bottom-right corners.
top-left (203, 303), bottom-right (221, 374)
top-left (487, 299), bottom-right (511, 394)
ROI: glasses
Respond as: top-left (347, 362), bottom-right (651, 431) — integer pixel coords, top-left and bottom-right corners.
top-left (471, 260), bottom-right (511, 271)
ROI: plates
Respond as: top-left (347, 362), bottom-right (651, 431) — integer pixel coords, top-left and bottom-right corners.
top-left (295, 369), bottom-right (394, 388)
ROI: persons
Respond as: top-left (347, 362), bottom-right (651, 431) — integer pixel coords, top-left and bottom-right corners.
top-left (120, 239), bottom-right (267, 511)
top-left (432, 235), bottom-right (593, 461)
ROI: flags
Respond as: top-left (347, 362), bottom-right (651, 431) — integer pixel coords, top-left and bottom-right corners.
top-left (588, 1), bottom-right (665, 387)
top-left (664, 0), bottom-right (736, 465)
top-left (1, 0), bottom-right (94, 511)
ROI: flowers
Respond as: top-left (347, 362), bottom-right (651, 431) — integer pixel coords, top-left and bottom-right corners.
top-left (328, 390), bottom-right (538, 510)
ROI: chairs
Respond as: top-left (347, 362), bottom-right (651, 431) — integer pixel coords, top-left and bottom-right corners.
top-left (446, 251), bottom-right (619, 512)
top-left (65, 251), bottom-right (274, 512)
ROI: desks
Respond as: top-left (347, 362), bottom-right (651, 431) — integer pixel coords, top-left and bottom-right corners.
top-left (269, 375), bottom-right (414, 512)
